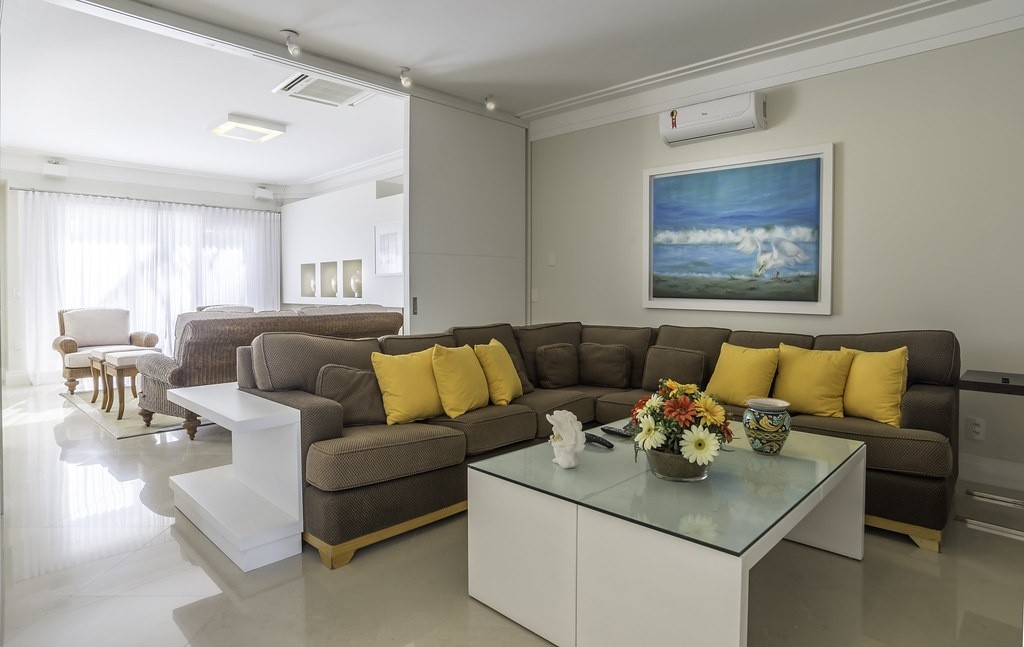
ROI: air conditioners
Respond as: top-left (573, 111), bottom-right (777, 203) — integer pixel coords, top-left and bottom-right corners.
top-left (658, 90), bottom-right (768, 144)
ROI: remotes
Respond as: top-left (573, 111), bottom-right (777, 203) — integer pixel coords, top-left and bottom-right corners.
top-left (601, 426), bottom-right (632, 438)
top-left (584, 432), bottom-right (614, 449)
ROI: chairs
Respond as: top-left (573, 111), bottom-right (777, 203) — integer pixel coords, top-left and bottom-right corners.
top-left (196, 304), bottom-right (255, 313)
top-left (51, 307), bottom-right (158, 397)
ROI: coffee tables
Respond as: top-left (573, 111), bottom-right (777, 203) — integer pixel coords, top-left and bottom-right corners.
top-left (468, 412), bottom-right (866, 647)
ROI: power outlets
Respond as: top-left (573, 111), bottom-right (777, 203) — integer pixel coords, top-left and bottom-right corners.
top-left (966, 416), bottom-right (986, 441)
top-left (532, 289), bottom-right (538, 302)
top-left (548, 250), bottom-right (556, 265)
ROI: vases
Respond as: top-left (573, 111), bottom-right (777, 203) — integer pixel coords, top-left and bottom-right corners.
top-left (644, 447), bottom-right (708, 483)
top-left (742, 397), bottom-right (791, 455)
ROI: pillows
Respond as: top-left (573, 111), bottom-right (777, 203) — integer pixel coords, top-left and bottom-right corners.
top-left (579, 342), bottom-right (632, 389)
top-left (536, 343), bottom-right (578, 390)
top-left (473, 339), bottom-right (524, 408)
top-left (369, 344), bottom-right (445, 425)
top-left (839, 345), bottom-right (910, 429)
top-left (315, 363), bottom-right (386, 429)
top-left (508, 354), bottom-right (534, 394)
top-left (772, 341), bottom-right (855, 418)
top-left (430, 343), bottom-right (490, 420)
top-left (641, 344), bottom-right (707, 393)
top-left (704, 342), bottom-right (780, 408)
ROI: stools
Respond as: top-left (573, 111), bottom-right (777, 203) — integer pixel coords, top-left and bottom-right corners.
top-left (104, 350), bottom-right (162, 420)
top-left (86, 346), bottom-right (141, 409)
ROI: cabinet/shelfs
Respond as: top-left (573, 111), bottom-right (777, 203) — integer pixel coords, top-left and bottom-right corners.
top-left (167, 380), bottom-right (304, 573)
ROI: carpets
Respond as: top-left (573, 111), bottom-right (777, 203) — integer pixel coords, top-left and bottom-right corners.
top-left (61, 385), bottom-right (215, 441)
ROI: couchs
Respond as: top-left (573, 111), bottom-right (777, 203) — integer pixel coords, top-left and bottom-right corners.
top-left (234, 323), bottom-right (961, 569)
top-left (133, 303), bottom-right (402, 441)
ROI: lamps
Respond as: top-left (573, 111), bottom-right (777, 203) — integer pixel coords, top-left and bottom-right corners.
top-left (255, 187), bottom-right (273, 202)
top-left (398, 66), bottom-right (412, 88)
top-left (39, 159), bottom-right (68, 180)
top-left (280, 30), bottom-right (301, 57)
top-left (207, 113), bottom-right (286, 144)
top-left (481, 93), bottom-right (496, 111)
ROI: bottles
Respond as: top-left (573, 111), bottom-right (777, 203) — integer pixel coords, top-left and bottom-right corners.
top-left (743, 397), bottom-right (792, 457)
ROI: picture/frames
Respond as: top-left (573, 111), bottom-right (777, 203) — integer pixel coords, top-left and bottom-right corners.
top-left (372, 218), bottom-right (405, 277)
top-left (641, 142), bottom-right (834, 316)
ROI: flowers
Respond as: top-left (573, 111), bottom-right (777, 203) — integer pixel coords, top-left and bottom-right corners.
top-left (624, 374), bottom-right (739, 465)
